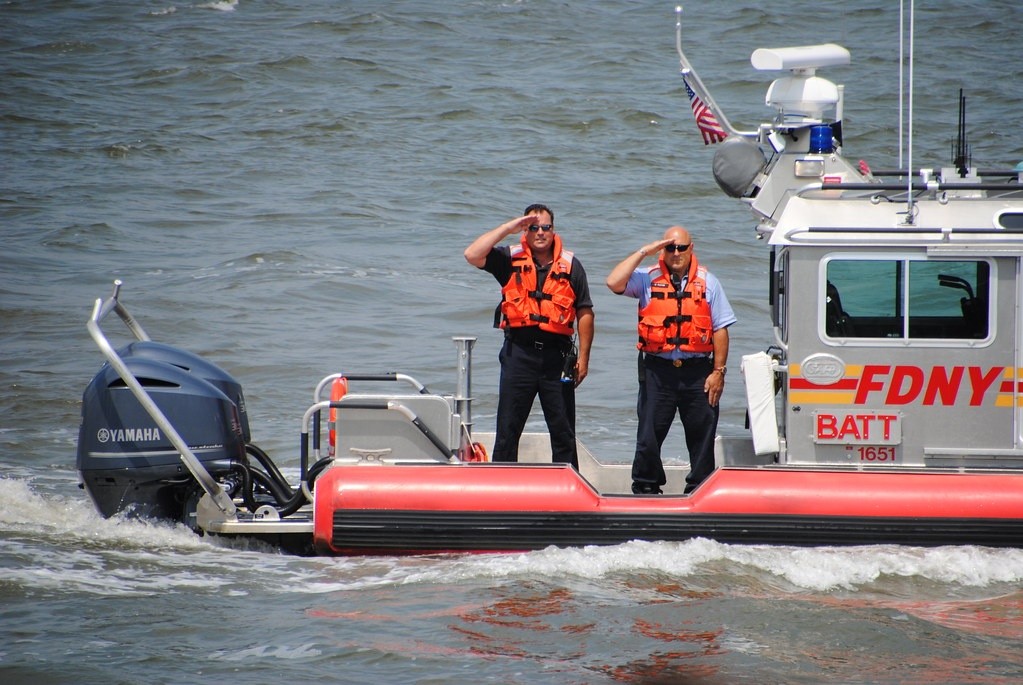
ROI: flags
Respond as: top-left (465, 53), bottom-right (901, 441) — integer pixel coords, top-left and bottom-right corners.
top-left (681, 81), bottom-right (727, 144)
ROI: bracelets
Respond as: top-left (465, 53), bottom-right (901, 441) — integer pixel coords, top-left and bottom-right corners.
top-left (639, 249), bottom-right (646, 255)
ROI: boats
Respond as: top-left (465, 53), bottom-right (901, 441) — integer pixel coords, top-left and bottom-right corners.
top-left (75, 0), bottom-right (1023, 552)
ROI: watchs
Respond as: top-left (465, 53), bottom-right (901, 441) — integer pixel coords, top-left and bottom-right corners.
top-left (714, 366), bottom-right (727, 375)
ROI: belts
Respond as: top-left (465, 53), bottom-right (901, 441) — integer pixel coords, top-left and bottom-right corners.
top-left (531, 341), bottom-right (544, 350)
top-left (645, 353), bottom-right (707, 367)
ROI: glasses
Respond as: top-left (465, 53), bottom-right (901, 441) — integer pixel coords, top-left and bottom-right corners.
top-left (528, 224), bottom-right (552, 232)
top-left (665, 244), bottom-right (690, 252)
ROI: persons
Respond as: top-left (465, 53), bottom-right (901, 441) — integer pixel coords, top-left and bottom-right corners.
top-left (605, 226), bottom-right (738, 494)
top-left (464, 204), bottom-right (593, 472)
